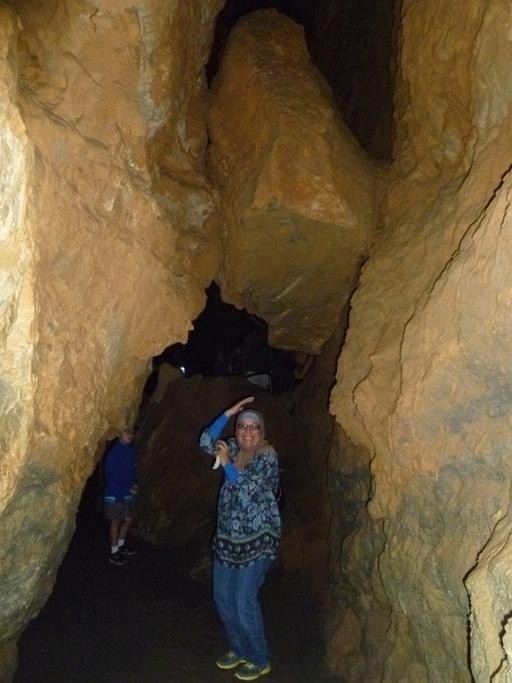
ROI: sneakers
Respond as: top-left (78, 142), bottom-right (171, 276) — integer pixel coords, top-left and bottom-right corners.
top-left (233, 662), bottom-right (271, 680)
top-left (215, 650), bottom-right (246, 669)
top-left (108, 545), bottom-right (137, 565)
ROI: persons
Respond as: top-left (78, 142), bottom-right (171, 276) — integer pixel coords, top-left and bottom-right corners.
top-left (102, 425), bottom-right (140, 566)
top-left (199, 396), bottom-right (282, 681)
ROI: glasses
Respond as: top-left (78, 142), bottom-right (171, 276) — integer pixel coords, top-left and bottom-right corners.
top-left (239, 423), bottom-right (260, 431)
